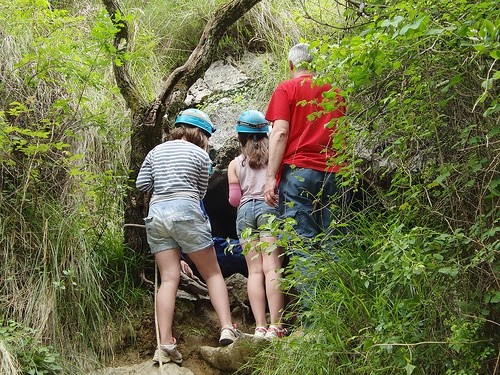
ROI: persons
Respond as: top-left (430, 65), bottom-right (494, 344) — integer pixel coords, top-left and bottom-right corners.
top-left (264, 43), bottom-right (346, 330)
top-left (179, 236), bottom-right (247, 278)
top-left (228, 111), bottom-right (286, 339)
top-left (136, 109), bottom-right (252, 362)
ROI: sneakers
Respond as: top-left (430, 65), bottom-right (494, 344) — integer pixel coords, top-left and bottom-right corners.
top-left (265, 324), bottom-right (288, 342)
top-left (219, 325), bottom-right (243, 346)
top-left (152, 331), bottom-right (184, 363)
top-left (254, 326), bottom-right (268, 339)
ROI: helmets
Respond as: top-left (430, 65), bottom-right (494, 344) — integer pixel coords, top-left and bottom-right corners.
top-left (236, 110), bottom-right (269, 133)
top-left (174, 109), bottom-right (212, 136)
